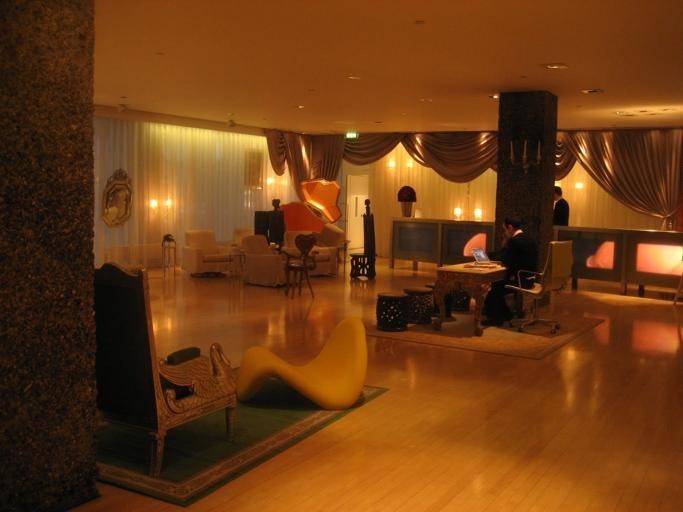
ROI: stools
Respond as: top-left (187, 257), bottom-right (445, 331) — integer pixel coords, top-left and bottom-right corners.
top-left (427, 283), bottom-right (469, 318)
top-left (404, 287), bottom-right (433, 325)
top-left (375, 292), bottom-right (409, 331)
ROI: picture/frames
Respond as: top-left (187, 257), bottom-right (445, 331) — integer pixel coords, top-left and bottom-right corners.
top-left (470, 247), bottom-right (491, 264)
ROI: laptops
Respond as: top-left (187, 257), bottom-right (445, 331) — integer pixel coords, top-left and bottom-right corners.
top-left (470, 248), bottom-right (502, 265)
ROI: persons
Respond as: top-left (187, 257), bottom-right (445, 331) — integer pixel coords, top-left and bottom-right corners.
top-left (553, 186), bottom-right (569, 226)
top-left (480, 214), bottom-right (539, 329)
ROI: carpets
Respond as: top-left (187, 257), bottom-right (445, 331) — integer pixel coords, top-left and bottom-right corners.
top-left (96, 365), bottom-right (389, 507)
top-left (365, 307), bottom-right (605, 359)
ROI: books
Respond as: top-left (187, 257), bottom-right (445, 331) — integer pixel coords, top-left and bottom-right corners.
top-left (464, 261), bottom-right (497, 268)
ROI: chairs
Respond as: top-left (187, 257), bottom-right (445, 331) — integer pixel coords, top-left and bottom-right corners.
top-left (93, 260), bottom-right (236, 477)
top-left (232, 228), bottom-right (255, 278)
top-left (505, 241), bottom-right (574, 333)
top-left (182, 230), bottom-right (231, 279)
top-left (285, 233), bottom-right (319, 299)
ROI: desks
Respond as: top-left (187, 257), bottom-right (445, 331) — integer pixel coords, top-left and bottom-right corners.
top-left (161, 233), bottom-right (179, 277)
top-left (436, 260), bottom-right (508, 337)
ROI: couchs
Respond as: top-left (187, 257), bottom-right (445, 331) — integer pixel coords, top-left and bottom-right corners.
top-left (283, 223), bottom-right (344, 277)
top-left (241, 235), bottom-right (288, 289)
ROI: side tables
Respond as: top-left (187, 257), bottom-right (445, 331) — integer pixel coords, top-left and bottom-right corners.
top-left (228, 253), bottom-right (245, 277)
top-left (349, 253), bottom-right (377, 279)
